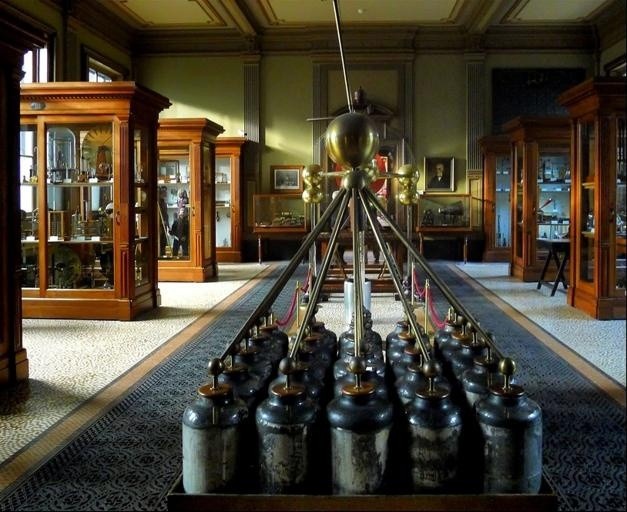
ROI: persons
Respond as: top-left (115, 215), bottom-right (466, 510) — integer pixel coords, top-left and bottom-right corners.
top-left (429, 162), bottom-right (450, 188)
top-left (159, 186), bottom-right (171, 256)
top-left (170, 206), bottom-right (189, 256)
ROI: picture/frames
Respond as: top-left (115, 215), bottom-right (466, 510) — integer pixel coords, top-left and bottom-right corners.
top-left (423, 155), bottom-right (455, 192)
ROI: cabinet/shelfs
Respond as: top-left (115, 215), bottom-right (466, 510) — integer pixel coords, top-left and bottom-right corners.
top-left (158, 115), bottom-right (227, 287)
top-left (215, 136), bottom-right (251, 263)
top-left (269, 164), bottom-right (304, 195)
top-left (475, 73), bottom-right (626, 320)
top-left (18, 78), bottom-right (173, 320)
